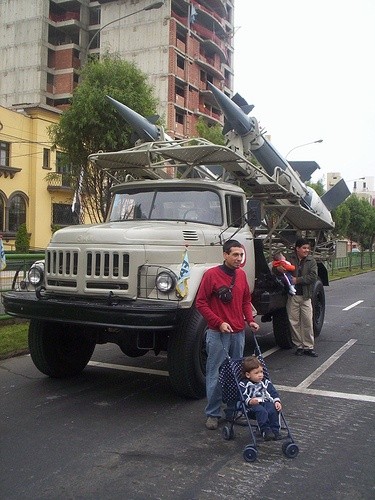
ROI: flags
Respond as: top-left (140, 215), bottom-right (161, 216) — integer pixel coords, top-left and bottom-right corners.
top-left (0, 237), bottom-right (7, 272)
top-left (176, 250), bottom-right (190, 296)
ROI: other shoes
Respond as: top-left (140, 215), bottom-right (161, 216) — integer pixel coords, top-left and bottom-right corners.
top-left (207, 417), bottom-right (218, 430)
top-left (305, 350), bottom-right (318, 357)
top-left (224, 415), bottom-right (248, 426)
top-left (264, 432), bottom-right (276, 441)
top-left (297, 349), bottom-right (303, 355)
top-left (274, 433), bottom-right (282, 441)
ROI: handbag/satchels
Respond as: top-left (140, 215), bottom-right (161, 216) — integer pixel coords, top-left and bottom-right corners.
top-left (217, 286), bottom-right (233, 302)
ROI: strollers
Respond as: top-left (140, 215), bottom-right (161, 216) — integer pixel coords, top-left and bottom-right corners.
top-left (218, 328), bottom-right (300, 463)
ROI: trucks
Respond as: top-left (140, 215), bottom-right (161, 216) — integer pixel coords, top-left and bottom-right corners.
top-left (0, 81), bottom-right (351, 403)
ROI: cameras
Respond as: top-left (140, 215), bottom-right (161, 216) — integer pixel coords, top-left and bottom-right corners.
top-left (218, 285), bottom-right (233, 304)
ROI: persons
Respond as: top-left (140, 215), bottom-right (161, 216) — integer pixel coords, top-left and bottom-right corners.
top-left (272, 252), bottom-right (297, 296)
top-left (238, 356), bottom-right (284, 440)
top-left (195, 240), bottom-right (260, 430)
top-left (272, 239), bottom-right (318, 357)
